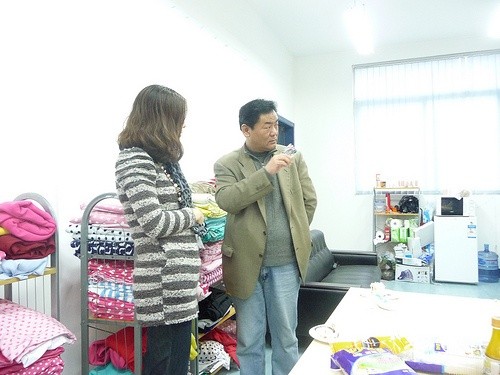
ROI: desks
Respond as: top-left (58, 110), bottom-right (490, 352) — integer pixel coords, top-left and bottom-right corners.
top-left (292, 287), bottom-right (500, 375)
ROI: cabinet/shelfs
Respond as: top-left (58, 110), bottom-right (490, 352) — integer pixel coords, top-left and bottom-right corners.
top-left (80, 192), bottom-right (236, 375)
top-left (0, 193), bottom-right (60, 375)
top-left (373, 187), bottom-right (420, 253)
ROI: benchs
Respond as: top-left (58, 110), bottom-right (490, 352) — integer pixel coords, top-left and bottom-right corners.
top-left (297, 229), bottom-right (381, 339)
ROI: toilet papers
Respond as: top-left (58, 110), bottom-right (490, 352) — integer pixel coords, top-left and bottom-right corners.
top-left (375, 231), bottom-right (385, 239)
top-left (404, 220), bottom-right (410, 228)
top-left (390, 219), bottom-right (403, 228)
top-left (412, 237), bottom-right (422, 258)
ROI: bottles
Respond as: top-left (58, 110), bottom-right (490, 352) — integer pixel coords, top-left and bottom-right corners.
top-left (481, 316), bottom-right (500, 375)
top-left (383, 224), bottom-right (391, 241)
top-left (477, 243), bottom-right (500, 283)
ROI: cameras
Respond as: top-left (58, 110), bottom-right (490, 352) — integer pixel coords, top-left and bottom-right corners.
top-left (282, 143), bottom-right (297, 157)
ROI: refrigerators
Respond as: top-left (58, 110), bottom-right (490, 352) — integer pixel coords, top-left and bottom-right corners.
top-left (433, 215), bottom-right (479, 283)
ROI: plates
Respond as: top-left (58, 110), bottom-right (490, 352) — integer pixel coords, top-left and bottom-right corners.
top-left (309, 324), bottom-right (350, 343)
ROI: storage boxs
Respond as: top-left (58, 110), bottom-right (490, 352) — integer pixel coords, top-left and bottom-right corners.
top-left (394, 263), bottom-right (431, 284)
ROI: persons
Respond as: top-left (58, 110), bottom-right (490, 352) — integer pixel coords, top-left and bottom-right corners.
top-left (115, 85), bottom-right (205, 375)
top-left (214, 99), bottom-right (317, 375)
